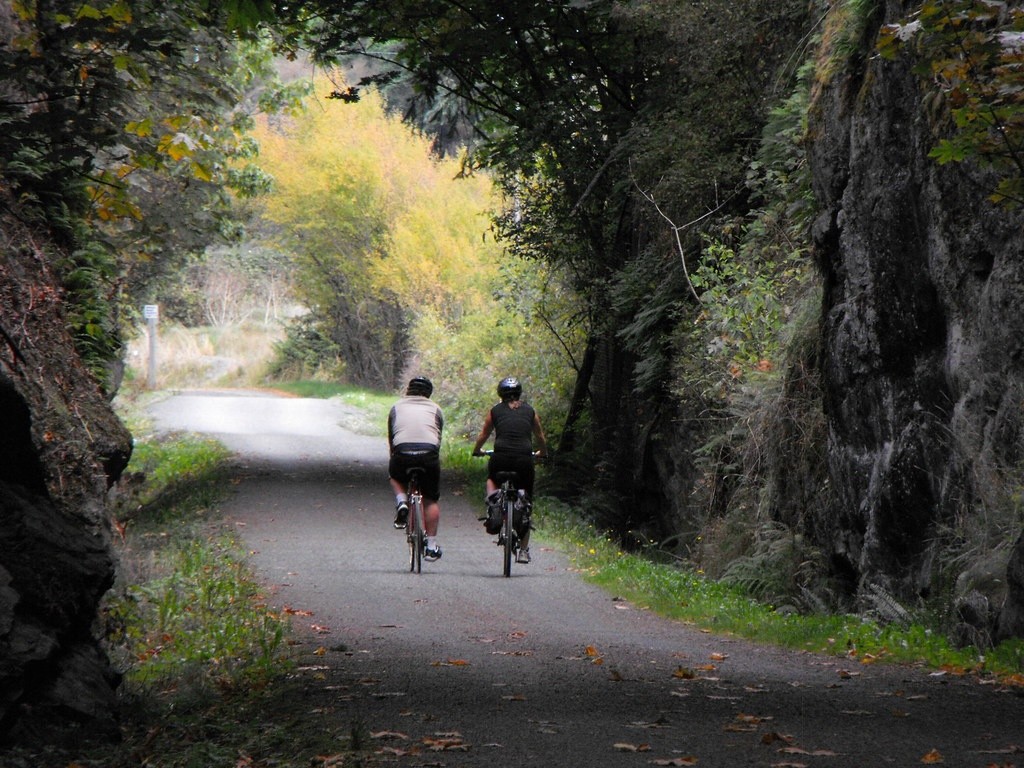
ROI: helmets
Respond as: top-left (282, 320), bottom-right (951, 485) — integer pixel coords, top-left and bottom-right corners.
top-left (407, 376), bottom-right (432, 398)
top-left (497, 376), bottom-right (522, 396)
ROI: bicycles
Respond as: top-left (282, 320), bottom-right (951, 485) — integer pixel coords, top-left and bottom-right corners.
top-left (395, 467), bottom-right (431, 574)
top-left (474, 448), bottom-right (548, 577)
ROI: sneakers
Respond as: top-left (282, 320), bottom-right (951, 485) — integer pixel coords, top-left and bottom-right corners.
top-left (393, 500), bottom-right (409, 529)
top-left (518, 547), bottom-right (532, 562)
top-left (425, 544), bottom-right (443, 562)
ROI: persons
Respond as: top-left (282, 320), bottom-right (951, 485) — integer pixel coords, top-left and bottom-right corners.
top-left (474, 376), bottom-right (546, 563)
top-left (388, 376), bottom-right (443, 536)
